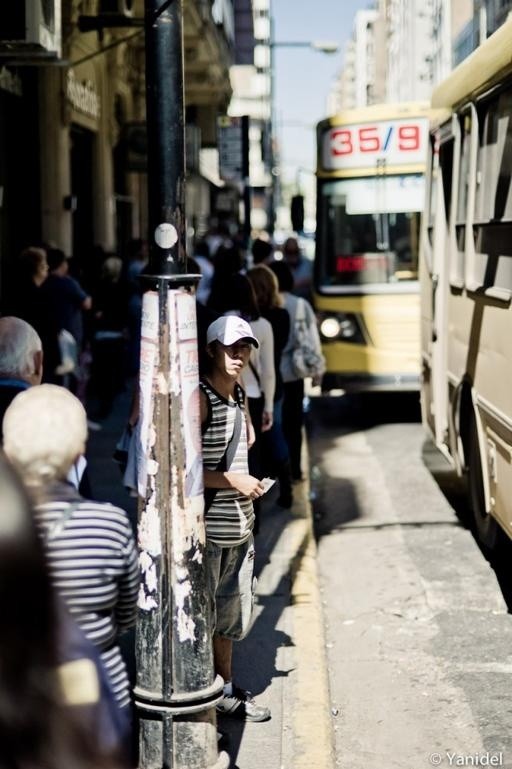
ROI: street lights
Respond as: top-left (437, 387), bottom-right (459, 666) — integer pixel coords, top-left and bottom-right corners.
top-left (239, 37), bottom-right (346, 233)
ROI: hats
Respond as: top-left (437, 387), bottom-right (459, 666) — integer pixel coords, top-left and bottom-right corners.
top-left (206, 315), bottom-right (260, 348)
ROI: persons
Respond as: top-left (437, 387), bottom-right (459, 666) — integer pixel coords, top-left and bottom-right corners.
top-left (0, 447), bottom-right (131, 768)
top-left (1, 381), bottom-right (141, 743)
top-left (198, 312), bottom-right (270, 740)
top-left (1, 222), bottom-right (328, 538)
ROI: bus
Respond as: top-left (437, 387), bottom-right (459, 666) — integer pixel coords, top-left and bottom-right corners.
top-left (408, 3), bottom-right (512, 559)
top-left (285, 98), bottom-right (423, 396)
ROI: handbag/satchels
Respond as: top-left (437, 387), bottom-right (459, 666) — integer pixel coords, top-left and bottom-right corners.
top-left (291, 348), bottom-right (327, 378)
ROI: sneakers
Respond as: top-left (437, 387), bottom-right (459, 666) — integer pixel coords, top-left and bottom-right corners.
top-left (218, 679), bottom-right (271, 722)
